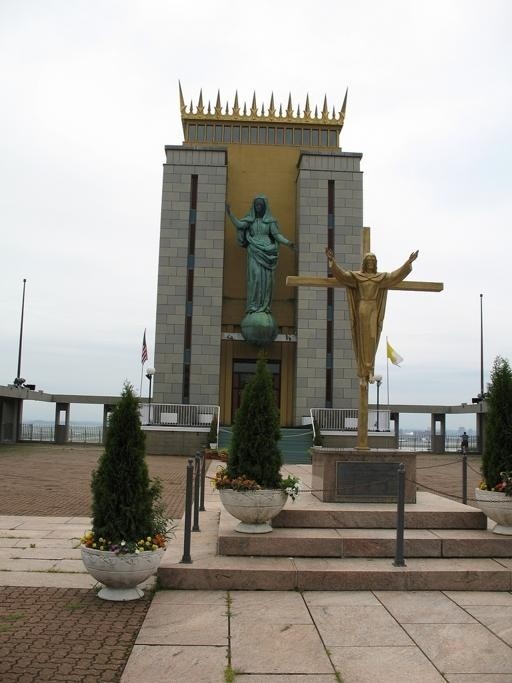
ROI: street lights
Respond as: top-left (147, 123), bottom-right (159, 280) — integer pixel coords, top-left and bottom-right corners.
top-left (146, 366), bottom-right (155, 425)
top-left (373, 375), bottom-right (382, 430)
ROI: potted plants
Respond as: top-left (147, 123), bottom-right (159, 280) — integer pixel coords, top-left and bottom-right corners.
top-left (79, 375), bottom-right (180, 602)
top-left (475, 351), bottom-right (512, 535)
top-left (208, 349), bottom-right (300, 534)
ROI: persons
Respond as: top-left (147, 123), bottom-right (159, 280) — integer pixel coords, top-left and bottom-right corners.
top-left (459, 431), bottom-right (468, 452)
top-left (227, 191), bottom-right (296, 312)
top-left (323, 246), bottom-right (419, 386)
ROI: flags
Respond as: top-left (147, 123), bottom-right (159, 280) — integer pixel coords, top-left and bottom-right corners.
top-left (386, 337), bottom-right (403, 367)
top-left (141, 329), bottom-right (149, 363)
